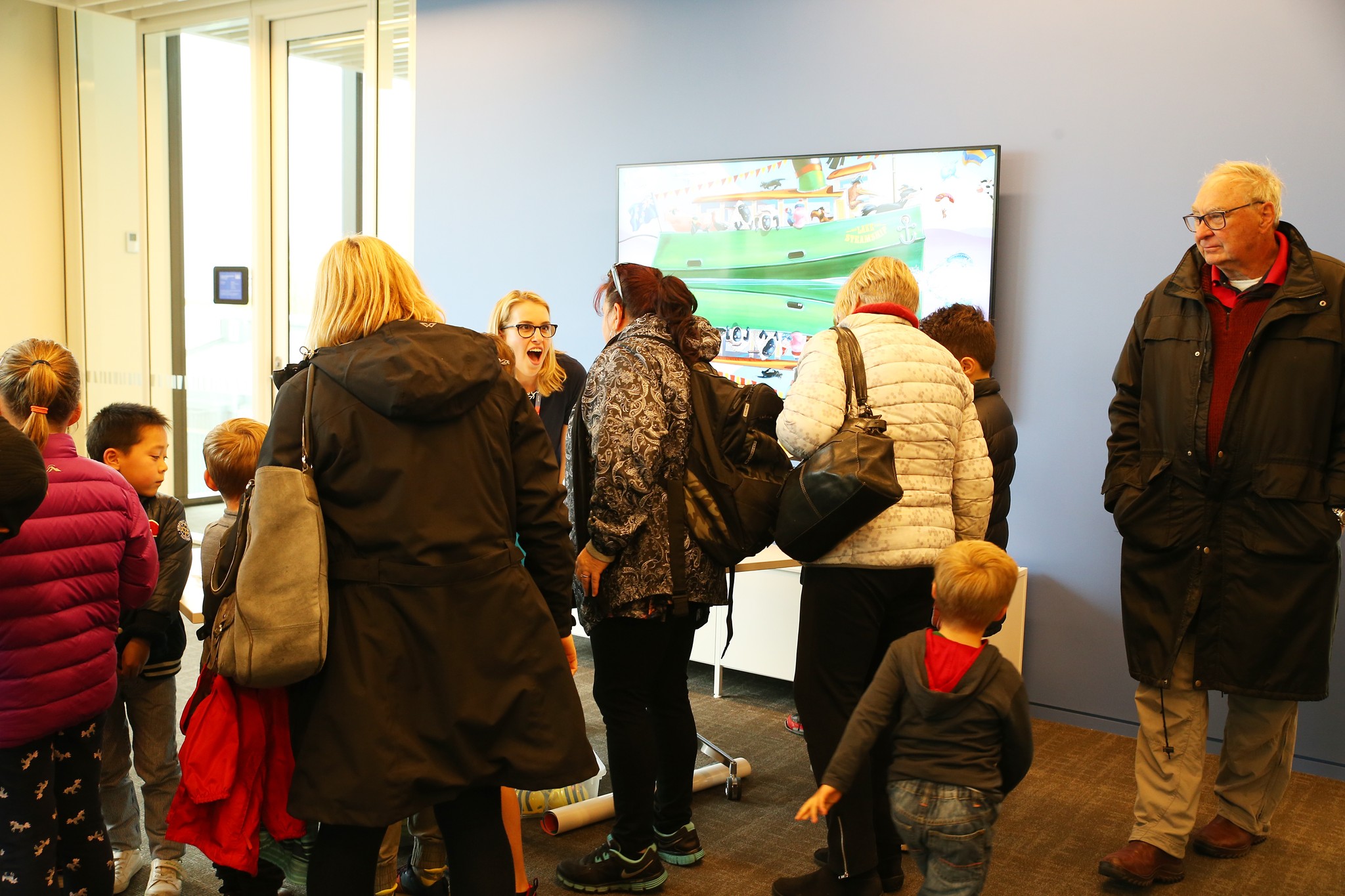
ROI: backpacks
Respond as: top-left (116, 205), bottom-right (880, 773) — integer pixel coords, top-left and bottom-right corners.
top-left (613, 333), bottom-right (794, 569)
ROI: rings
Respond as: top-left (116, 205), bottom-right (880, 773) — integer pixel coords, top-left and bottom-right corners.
top-left (580, 573), bottom-right (590, 578)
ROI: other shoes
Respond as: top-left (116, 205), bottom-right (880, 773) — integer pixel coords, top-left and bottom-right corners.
top-left (815, 846), bottom-right (828, 865)
top-left (515, 876), bottom-right (538, 896)
top-left (771, 868), bottom-right (884, 896)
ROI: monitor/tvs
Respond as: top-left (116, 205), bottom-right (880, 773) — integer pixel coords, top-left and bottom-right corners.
top-left (615, 144), bottom-right (1001, 398)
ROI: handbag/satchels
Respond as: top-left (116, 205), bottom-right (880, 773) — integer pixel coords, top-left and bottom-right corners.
top-left (771, 325), bottom-right (904, 563)
top-left (210, 359), bottom-right (330, 689)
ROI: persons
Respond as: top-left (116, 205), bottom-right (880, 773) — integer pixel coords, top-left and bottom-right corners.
top-left (0, 336), bottom-right (160, 896)
top-left (794, 538), bottom-right (1034, 896)
top-left (895, 302), bottom-right (1018, 852)
top-left (199, 236), bottom-right (600, 896)
top-left (85, 402), bottom-right (193, 896)
top-left (555, 263), bottom-right (722, 894)
top-left (489, 290), bottom-right (588, 487)
top-left (1099, 162), bottom-right (1345, 888)
top-left (770, 256), bottom-right (995, 896)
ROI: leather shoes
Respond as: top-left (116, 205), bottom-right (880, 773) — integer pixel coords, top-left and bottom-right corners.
top-left (1097, 840), bottom-right (1186, 891)
top-left (1193, 812), bottom-right (1268, 857)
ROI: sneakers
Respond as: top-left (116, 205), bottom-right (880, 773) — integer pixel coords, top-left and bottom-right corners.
top-left (606, 821), bottom-right (705, 865)
top-left (145, 856), bottom-right (186, 896)
top-left (113, 846), bottom-right (143, 893)
top-left (394, 859), bottom-right (450, 896)
top-left (556, 833), bottom-right (668, 892)
top-left (785, 713), bottom-right (805, 735)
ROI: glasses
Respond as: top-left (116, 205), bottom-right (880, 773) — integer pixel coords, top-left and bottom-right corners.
top-left (607, 261), bottom-right (625, 319)
top-left (501, 323), bottom-right (559, 338)
top-left (1183, 201), bottom-right (1265, 233)
